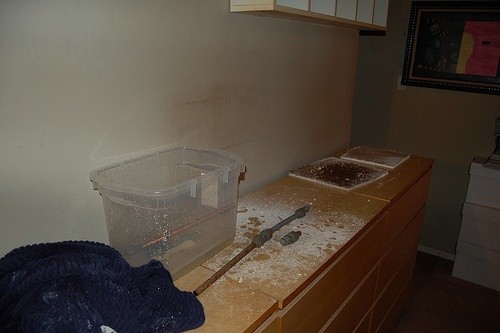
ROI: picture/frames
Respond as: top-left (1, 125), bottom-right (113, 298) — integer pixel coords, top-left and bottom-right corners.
top-left (400, 1), bottom-right (500, 95)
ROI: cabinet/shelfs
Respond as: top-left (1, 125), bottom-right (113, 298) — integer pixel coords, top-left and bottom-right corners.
top-left (114, 144), bottom-right (434, 333)
top-left (229, 0), bottom-right (389, 37)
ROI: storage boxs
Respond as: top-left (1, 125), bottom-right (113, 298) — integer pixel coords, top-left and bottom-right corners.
top-left (88, 145), bottom-right (244, 281)
